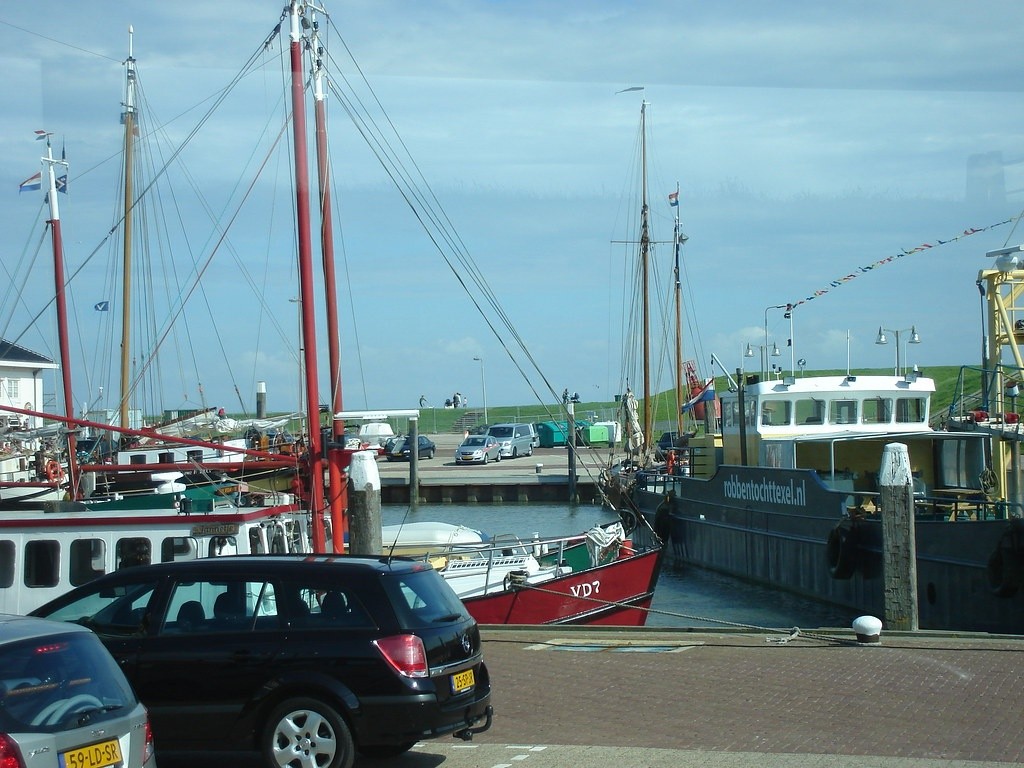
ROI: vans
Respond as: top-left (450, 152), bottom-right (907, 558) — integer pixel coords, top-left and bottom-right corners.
top-left (482, 423), bottom-right (535, 459)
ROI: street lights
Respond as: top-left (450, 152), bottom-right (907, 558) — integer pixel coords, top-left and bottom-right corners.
top-left (473, 357), bottom-right (488, 425)
top-left (764, 304), bottom-right (788, 382)
top-left (744, 342), bottom-right (783, 381)
top-left (874, 324), bottom-right (922, 377)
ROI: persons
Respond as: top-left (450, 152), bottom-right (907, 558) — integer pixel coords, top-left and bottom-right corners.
top-left (562, 389), bottom-right (569, 404)
top-left (453, 393), bottom-right (462, 408)
top-left (419, 395), bottom-right (426, 407)
top-left (463, 397), bottom-right (467, 407)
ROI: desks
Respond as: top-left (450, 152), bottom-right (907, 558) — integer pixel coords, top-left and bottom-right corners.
top-left (932, 488), bottom-right (992, 521)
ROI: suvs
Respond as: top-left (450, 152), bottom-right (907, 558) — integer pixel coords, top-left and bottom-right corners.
top-left (26, 550), bottom-right (496, 768)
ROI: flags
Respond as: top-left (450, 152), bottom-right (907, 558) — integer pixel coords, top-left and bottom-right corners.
top-left (56, 176), bottom-right (67, 193)
top-left (681, 380), bottom-right (716, 414)
top-left (121, 112), bottom-right (138, 124)
top-left (19, 172), bottom-right (42, 194)
top-left (94, 301), bottom-right (109, 311)
top-left (669, 193), bottom-right (678, 206)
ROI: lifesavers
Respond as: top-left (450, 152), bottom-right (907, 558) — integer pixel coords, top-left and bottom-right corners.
top-left (666, 453), bottom-right (675, 473)
top-left (46, 461), bottom-right (63, 483)
top-left (825, 526), bottom-right (859, 580)
top-left (985, 548), bottom-right (1020, 598)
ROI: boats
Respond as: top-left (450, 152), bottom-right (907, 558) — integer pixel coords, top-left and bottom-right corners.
top-left (595, 79), bottom-right (1023, 637)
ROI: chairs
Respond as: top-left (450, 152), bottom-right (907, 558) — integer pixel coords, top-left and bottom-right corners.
top-left (316, 591), bottom-right (348, 630)
top-left (480, 440), bottom-right (488, 446)
top-left (470, 439), bottom-right (477, 446)
top-left (169, 600), bottom-right (205, 635)
top-left (31, 657), bottom-right (69, 684)
top-left (805, 417), bottom-right (822, 424)
top-left (506, 428), bottom-right (513, 435)
top-left (211, 593), bottom-right (240, 631)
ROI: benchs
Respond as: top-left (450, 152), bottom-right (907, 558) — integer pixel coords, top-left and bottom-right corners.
top-left (916, 502), bottom-right (961, 508)
top-left (972, 502), bottom-right (996, 507)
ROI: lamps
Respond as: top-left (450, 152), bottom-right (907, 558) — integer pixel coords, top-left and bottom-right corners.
top-left (905, 374), bottom-right (917, 384)
top-left (783, 376), bottom-right (795, 386)
top-left (912, 363), bottom-right (919, 375)
top-left (727, 387), bottom-right (736, 393)
top-left (798, 358), bottom-right (807, 377)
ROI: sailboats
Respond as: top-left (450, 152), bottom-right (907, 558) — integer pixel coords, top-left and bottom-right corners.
top-left (0, 0), bottom-right (669, 629)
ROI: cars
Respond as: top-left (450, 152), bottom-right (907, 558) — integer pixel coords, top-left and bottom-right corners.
top-left (454, 434), bottom-right (502, 465)
top-left (385, 436), bottom-right (436, 462)
top-left (654, 431), bottom-right (688, 461)
top-left (1, 613), bottom-right (158, 768)
